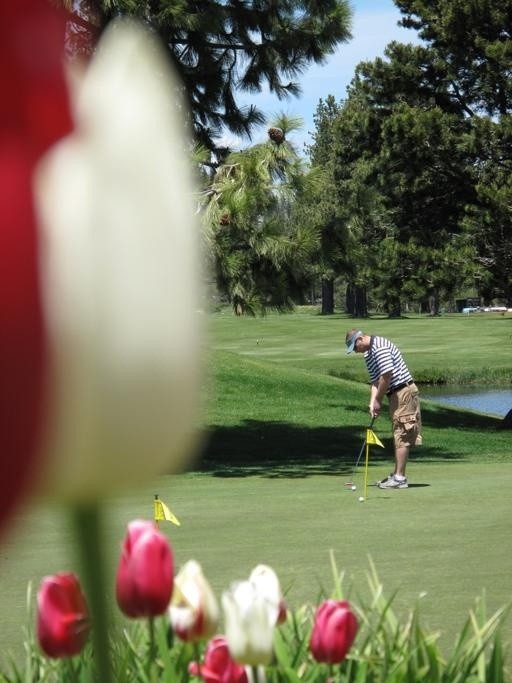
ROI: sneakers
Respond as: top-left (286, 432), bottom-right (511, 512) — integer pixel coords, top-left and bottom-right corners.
top-left (375, 473), bottom-right (396, 487)
top-left (379, 474), bottom-right (409, 490)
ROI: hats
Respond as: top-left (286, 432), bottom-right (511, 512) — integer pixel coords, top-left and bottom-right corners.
top-left (345, 330), bottom-right (363, 354)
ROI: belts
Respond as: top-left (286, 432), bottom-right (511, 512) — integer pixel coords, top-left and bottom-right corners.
top-left (386, 379), bottom-right (413, 397)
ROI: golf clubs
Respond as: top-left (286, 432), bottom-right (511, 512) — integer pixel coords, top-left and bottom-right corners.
top-left (344, 414), bottom-right (376, 489)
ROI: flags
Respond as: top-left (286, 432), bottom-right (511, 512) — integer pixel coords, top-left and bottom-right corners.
top-left (367, 430), bottom-right (386, 449)
top-left (155, 500), bottom-right (180, 527)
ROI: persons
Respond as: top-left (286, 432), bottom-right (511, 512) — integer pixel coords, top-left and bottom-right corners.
top-left (347, 328), bottom-right (423, 489)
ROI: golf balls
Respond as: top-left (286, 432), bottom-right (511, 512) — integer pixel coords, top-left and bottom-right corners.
top-left (352, 485), bottom-right (356, 489)
top-left (360, 497), bottom-right (364, 502)
